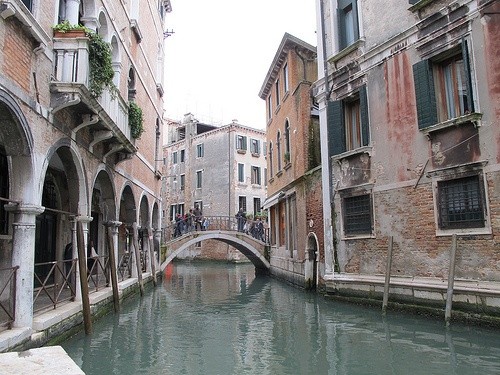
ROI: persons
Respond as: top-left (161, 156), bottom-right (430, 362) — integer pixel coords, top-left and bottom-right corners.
top-left (168, 207), bottom-right (209, 239)
top-left (63, 243), bottom-right (73, 289)
top-left (231, 216), bottom-right (269, 243)
top-left (235, 208), bottom-right (247, 231)
top-left (88, 233), bottom-right (99, 288)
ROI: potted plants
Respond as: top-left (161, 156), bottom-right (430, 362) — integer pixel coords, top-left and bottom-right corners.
top-left (53, 20), bottom-right (89, 38)
